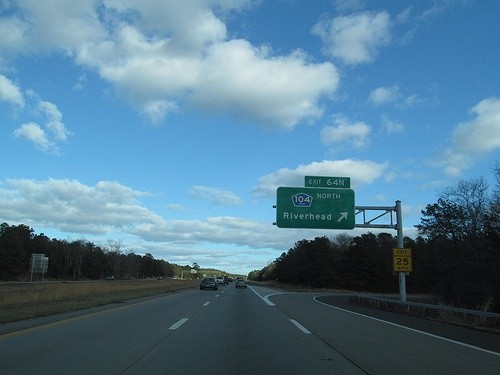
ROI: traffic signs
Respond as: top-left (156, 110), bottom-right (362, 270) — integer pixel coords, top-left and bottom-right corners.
top-left (276, 186), bottom-right (357, 230)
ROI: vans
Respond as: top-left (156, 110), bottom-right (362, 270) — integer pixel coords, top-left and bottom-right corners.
top-left (235, 276), bottom-right (244, 281)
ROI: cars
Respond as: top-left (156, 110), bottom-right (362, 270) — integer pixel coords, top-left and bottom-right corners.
top-left (171, 276), bottom-right (177, 280)
top-left (157, 276), bottom-right (164, 280)
top-left (200, 277), bottom-right (219, 291)
top-left (235, 279), bottom-right (248, 288)
top-left (105, 276), bottom-right (116, 281)
top-left (214, 275), bottom-right (233, 286)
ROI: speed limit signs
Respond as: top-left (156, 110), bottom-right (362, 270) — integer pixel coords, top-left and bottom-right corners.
top-left (393, 256), bottom-right (412, 273)
